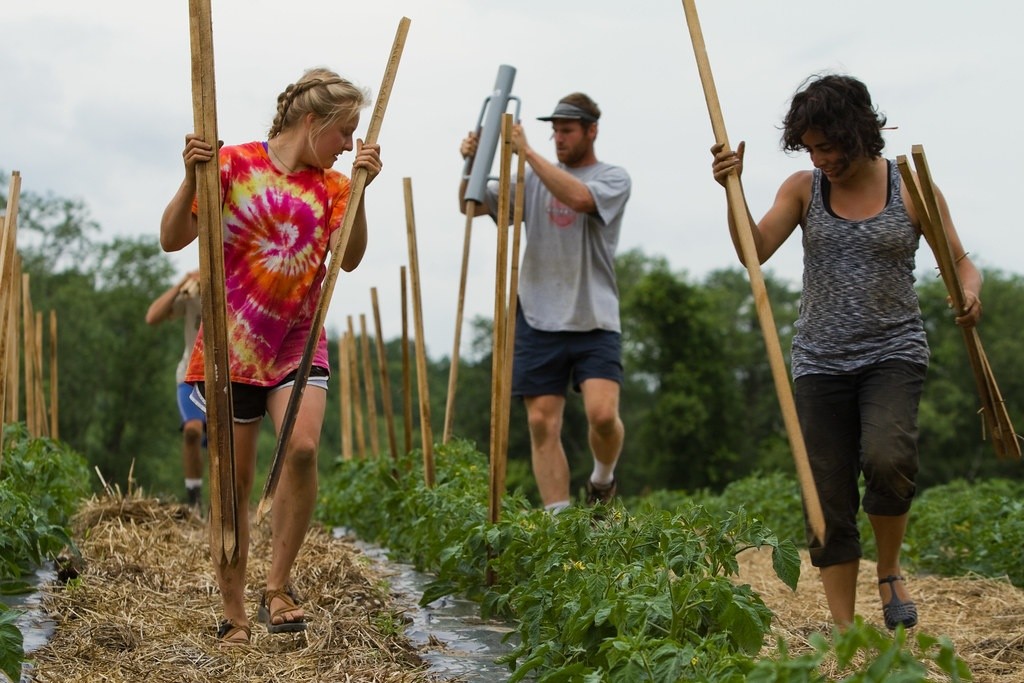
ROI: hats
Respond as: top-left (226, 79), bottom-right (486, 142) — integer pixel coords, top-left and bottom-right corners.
top-left (537, 102), bottom-right (596, 123)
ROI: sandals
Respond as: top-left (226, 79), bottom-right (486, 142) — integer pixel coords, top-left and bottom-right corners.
top-left (217, 616), bottom-right (251, 644)
top-left (877, 575), bottom-right (917, 629)
top-left (258, 586), bottom-right (306, 633)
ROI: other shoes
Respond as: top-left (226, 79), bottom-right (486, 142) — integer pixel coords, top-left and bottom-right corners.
top-left (186, 488), bottom-right (203, 519)
top-left (585, 479), bottom-right (615, 528)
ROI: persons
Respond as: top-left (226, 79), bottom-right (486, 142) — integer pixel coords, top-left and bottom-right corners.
top-left (711, 77), bottom-right (982, 640)
top-left (145, 69), bottom-right (384, 648)
top-left (460, 93), bottom-right (630, 524)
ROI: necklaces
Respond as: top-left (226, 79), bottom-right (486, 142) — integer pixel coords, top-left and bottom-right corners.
top-left (267, 142), bottom-right (295, 172)
